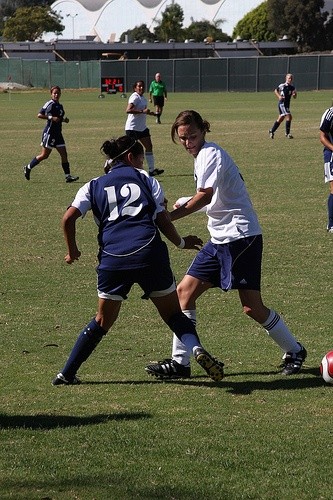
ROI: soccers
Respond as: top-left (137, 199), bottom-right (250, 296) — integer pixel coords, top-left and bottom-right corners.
top-left (319, 349), bottom-right (333, 385)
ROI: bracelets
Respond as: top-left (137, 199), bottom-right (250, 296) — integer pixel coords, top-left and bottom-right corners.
top-left (177, 238), bottom-right (185, 248)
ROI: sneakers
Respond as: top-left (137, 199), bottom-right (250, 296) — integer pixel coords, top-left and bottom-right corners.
top-left (194, 348), bottom-right (225, 382)
top-left (49, 372), bottom-right (82, 386)
top-left (145, 358), bottom-right (191, 378)
top-left (279, 342), bottom-right (307, 375)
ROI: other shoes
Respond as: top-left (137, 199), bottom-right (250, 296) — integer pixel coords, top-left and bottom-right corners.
top-left (66, 175), bottom-right (79, 183)
top-left (268, 129), bottom-right (275, 140)
top-left (156, 119), bottom-right (161, 124)
top-left (23, 165), bottom-right (31, 181)
top-left (104, 159), bottom-right (112, 174)
top-left (326, 225), bottom-right (333, 233)
top-left (285, 133), bottom-right (293, 139)
top-left (149, 168), bottom-right (165, 177)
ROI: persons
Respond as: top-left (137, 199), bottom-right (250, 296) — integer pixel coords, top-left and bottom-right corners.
top-left (268, 73), bottom-right (297, 140)
top-left (319, 101), bottom-right (333, 234)
top-left (103, 80), bottom-right (164, 177)
top-left (146, 111), bottom-right (308, 378)
top-left (23, 86), bottom-right (79, 183)
top-left (149, 73), bottom-right (168, 124)
top-left (53, 136), bottom-right (225, 385)
